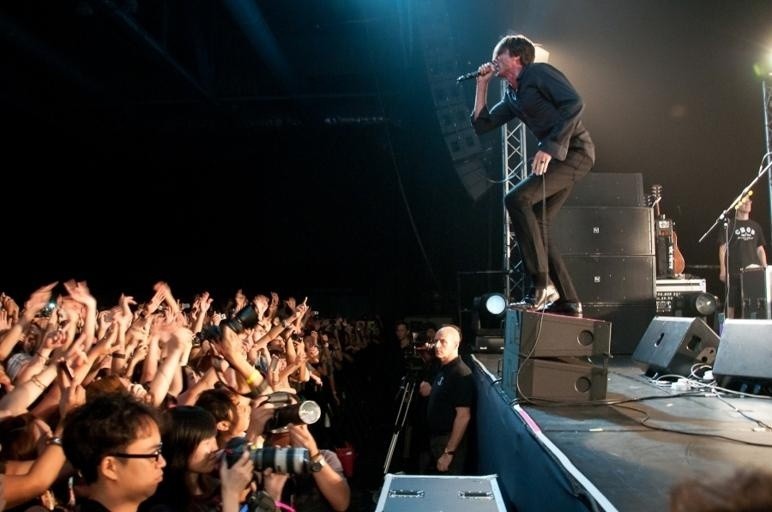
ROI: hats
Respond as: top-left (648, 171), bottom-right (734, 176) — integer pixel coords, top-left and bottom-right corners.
top-left (7, 353), bottom-right (32, 382)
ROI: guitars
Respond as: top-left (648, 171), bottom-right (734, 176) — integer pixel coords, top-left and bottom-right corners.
top-left (649, 184), bottom-right (686, 276)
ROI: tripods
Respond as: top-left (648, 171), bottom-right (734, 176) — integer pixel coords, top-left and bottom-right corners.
top-left (380, 381), bottom-right (418, 474)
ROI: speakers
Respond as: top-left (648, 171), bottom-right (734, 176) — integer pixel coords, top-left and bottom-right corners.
top-left (549, 172), bottom-right (657, 355)
top-left (632, 315), bottom-right (720, 380)
top-left (413, 0), bottom-right (501, 202)
top-left (712, 318), bottom-right (772, 396)
top-left (500, 305), bottom-right (612, 404)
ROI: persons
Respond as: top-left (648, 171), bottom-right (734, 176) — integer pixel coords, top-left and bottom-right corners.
top-left (718, 191), bottom-right (769, 319)
top-left (468, 34), bottom-right (597, 319)
top-left (422, 324), bottom-right (476, 476)
top-left (0, 277), bottom-right (436, 510)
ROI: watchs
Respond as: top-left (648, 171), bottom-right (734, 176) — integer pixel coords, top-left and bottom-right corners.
top-left (444, 448), bottom-right (457, 456)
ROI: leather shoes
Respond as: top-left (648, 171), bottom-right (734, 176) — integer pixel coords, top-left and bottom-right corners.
top-left (538, 296), bottom-right (582, 317)
top-left (509, 283), bottom-right (560, 312)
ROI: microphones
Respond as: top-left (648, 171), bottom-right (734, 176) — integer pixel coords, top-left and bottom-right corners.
top-left (455, 64), bottom-right (497, 83)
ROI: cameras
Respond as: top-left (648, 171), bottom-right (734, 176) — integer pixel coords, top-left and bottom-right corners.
top-left (225, 437), bottom-right (321, 477)
top-left (61, 361), bottom-right (73, 383)
top-left (238, 490), bottom-right (281, 512)
top-left (405, 355), bottom-right (427, 380)
top-left (314, 311), bottom-right (319, 315)
top-left (196, 303), bottom-right (259, 340)
top-left (40, 303), bottom-right (58, 315)
top-left (266, 399), bottom-right (322, 435)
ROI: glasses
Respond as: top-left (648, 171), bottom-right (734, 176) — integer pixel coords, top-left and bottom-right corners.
top-left (109, 448), bottom-right (161, 463)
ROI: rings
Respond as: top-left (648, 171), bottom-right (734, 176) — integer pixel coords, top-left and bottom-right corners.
top-left (539, 158), bottom-right (548, 164)
